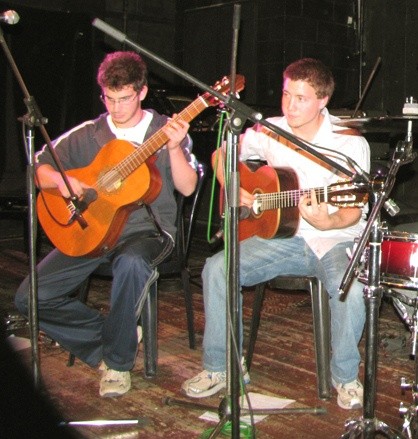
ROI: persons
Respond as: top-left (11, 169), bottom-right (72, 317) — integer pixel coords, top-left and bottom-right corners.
top-left (15, 52), bottom-right (197, 396)
top-left (181, 57), bottom-right (371, 410)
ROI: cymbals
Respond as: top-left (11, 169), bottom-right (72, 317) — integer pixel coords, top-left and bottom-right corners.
top-left (333, 115), bottom-right (418, 131)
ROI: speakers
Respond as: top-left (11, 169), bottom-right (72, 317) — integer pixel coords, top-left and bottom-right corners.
top-left (180, 123), bottom-right (228, 253)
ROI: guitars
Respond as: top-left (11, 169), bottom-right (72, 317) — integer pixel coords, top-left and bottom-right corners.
top-left (220, 159), bottom-right (368, 244)
top-left (36, 73), bottom-right (246, 258)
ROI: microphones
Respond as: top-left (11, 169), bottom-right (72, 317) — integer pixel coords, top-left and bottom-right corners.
top-left (0, 10), bottom-right (20, 24)
top-left (347, 157), bottom-right (400, 216)
top-left (68, 188), bottom-right (99, 225)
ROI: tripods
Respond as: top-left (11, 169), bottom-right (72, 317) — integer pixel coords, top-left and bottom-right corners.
top-left (165, 0), bottom-right (326, 439)
top-left (337, 118), bottom-right (413, 439)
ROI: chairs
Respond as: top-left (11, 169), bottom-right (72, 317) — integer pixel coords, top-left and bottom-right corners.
top-left (65, 162), bottom-right (206, 377)
top-left (244, 275), bottom-right (330, 401)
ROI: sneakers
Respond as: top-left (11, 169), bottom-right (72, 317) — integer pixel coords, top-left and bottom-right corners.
top-left (182, 357), bottom-right (250, 397)
top-left (98, 365), bottom-right (130, 396)
top-left (97, 328), bottom-right (142, 370)
top-left (331, 378), bottom-right (364, 409)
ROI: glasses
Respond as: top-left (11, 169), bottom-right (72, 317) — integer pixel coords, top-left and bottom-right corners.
top-left (101, 92), bottom-right (139, 105)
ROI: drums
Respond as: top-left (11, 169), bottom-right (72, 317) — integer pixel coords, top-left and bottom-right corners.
top-left (356, 226), bottom-right (418, 293)
